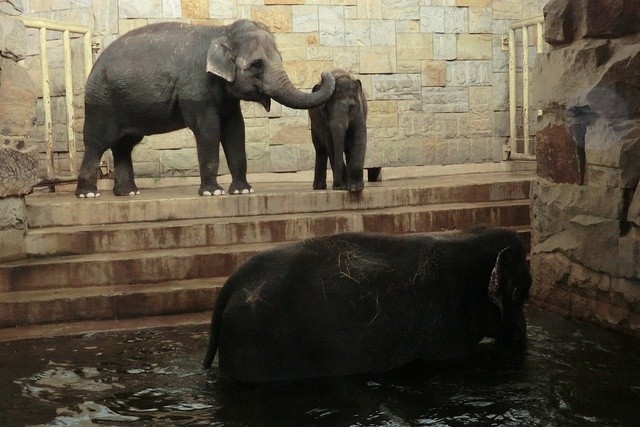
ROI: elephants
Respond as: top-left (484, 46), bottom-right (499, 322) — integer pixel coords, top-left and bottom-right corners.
top-left (203, 223), bottom-right (535, 393)
top-left (307, 69), bottom-right (372, 193)
top-left (74, 18), bottom-right (335, 196)
